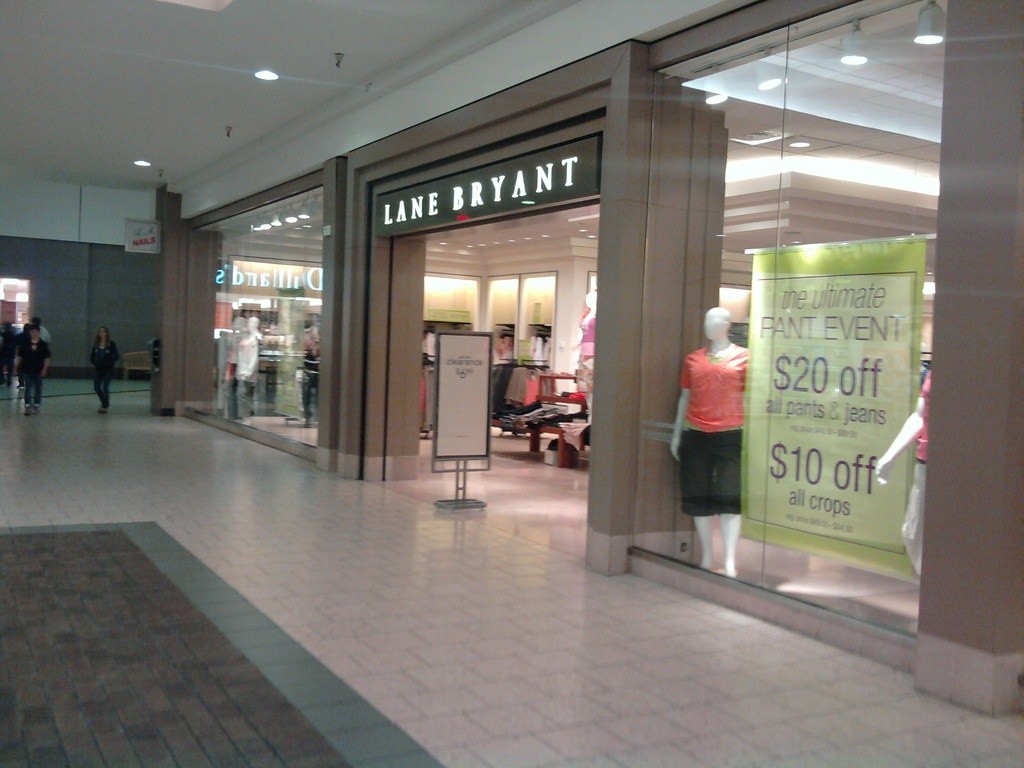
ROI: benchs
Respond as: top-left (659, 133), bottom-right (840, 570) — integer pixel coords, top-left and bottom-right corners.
top-left (123, 350), bottom-right (151, 383)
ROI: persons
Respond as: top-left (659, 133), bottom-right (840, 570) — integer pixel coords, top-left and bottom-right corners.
top-left (669, 307), bottom-right (756, 579)
top-left (576, 290), bottom-right (601, 426)
top-left (874, 367), bottom-right (935, 576)
top-left (90, 327), bottom-right (118, 414)
top-left (234, 317), bottom-right (263, 426)
top-left (0, 316), bottom-right (51, 415)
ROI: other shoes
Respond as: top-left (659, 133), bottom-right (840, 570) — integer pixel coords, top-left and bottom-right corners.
top-left (0, 379), bottom-right (12, 386)
top-left (24, 407), bottom-right (30, 415)
top-left (32, 406), bottom-right (40, 415)
top-left (98, 406), bottom-right (108, 413)
top-left (16, 384), bottom-right (25, 389)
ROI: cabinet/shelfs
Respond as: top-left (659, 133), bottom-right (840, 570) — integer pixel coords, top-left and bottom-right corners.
top-left (530, 372), bottom-right (591, 470)
